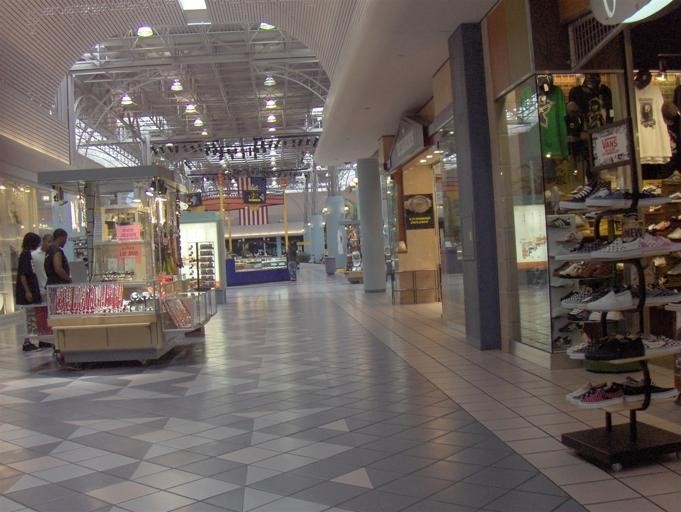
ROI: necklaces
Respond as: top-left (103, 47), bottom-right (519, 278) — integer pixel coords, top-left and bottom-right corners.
top-left (48, 284), bottom-right (123, 316)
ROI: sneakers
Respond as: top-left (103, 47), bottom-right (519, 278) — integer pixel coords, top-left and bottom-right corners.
top-left (549, 182), bottom-right (681, 360)
top-left (566, 376), bottom-right (677, 408)
top-left (23, 344), bottom-right (37, 351)
top-left (39, 341), bottom-right (52, 347)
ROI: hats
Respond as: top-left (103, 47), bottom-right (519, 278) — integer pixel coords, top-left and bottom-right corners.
top-left (663, 102), bottom-right (679, 125)
top-left (634, 72), bottom-right (651, 88)
top-left (538, 74), bottom-right (553, 92)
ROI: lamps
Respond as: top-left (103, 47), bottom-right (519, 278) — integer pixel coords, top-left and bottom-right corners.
top-left (121, 26), bottom-right (211, 137)
top-left (258, 22), bottom-right (282, 138)
top-left (145, 136), bottom-right (319, 160)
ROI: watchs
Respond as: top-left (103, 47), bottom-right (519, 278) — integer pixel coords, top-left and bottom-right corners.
top-left (404, 196), bottom-right (432, 213)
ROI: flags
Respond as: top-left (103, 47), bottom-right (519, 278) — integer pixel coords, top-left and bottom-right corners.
top-left (237, 175), bottom-right (268, 225)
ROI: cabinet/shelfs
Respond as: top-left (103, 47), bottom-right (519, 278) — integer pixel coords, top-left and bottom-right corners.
top-left (46, 177), bottom-right (218, 370)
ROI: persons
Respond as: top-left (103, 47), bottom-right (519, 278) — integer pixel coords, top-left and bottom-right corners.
top-left (44, 228), bottom-right (72, 289)
top-left (30, 234), bottom-right (53, 347)
top-left (287, 244), bottom-right (297, 281)
top-left (16, 232), bottom-right (42, 351)
